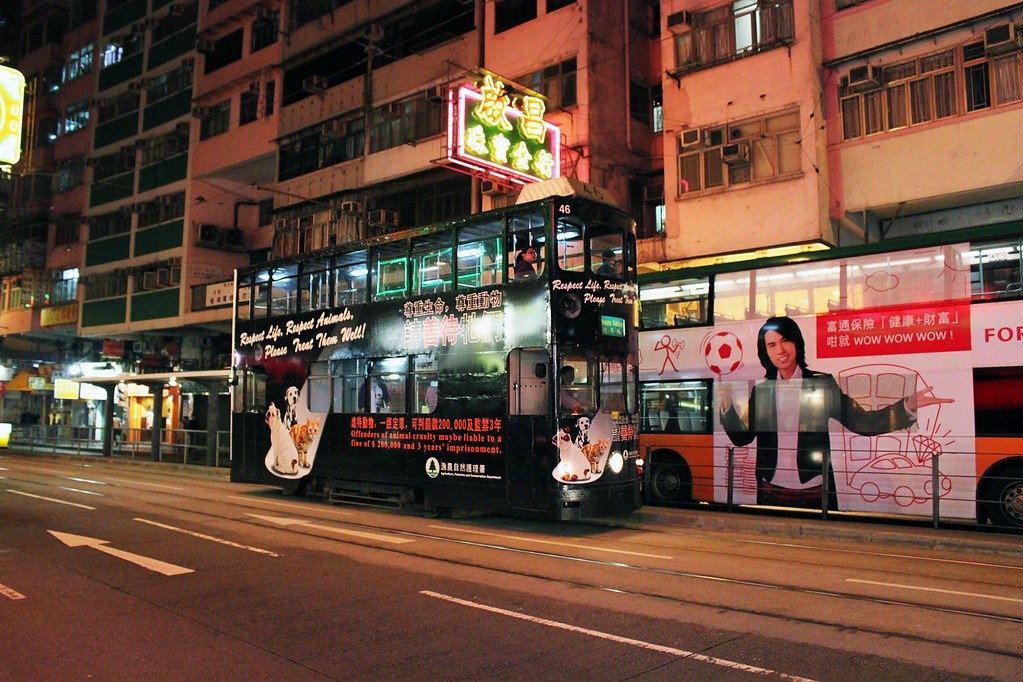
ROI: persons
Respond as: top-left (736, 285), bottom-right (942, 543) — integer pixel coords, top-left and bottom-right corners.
top-left (180, 413), bottom-right (199, 452)
top-left (718, 317), bottom-right (955, 510)
top-left (513, 245), bottom-right (538, 282)
top-left (20, 407), bottom-right (62, 445)
top-left (113, 411), bottom-right (124, 447)
top-left (560, 365), bottom-right (597, 415)
top-left (597, 250), bottom-right (624, 280)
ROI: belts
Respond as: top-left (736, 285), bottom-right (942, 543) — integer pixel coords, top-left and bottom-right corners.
top-left (758, 482), bottom-right (823, 500)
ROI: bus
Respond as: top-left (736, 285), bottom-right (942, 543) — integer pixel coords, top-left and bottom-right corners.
top-left (563, 220), bottom-right (1023, 534)
top-left (229, 177), bottom-right (642, 523)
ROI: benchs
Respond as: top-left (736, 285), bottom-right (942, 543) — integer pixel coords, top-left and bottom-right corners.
top-left (342, 282), bottom-right (477, 306)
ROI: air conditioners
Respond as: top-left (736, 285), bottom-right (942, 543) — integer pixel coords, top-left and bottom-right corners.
top-left (480, 180), bottom-right (507, 197)
top-left (983, 21), bottom-right (1023, 57)
top-left (140, 78), bottom-right (151, 89)
top-left (131, 24), bottom-right (145, 37)
top-left (109, 36), bottom-right (123, 45)
top-left (342, 202), bottom-right (362, 214)
top-left (322, 120), bottom-right (346, 138)
top-left (681, 127), bottom-right (712, 148)
top-left (425, 85), bottom-right (447, 106)
top-left (720, 144), bottom-right (750, 163)
top-left (275, 218), bottom-right (288, 232)
top-left (195, 39), bottom-right (209, 53)
top-left (248, 82), bottom-right (260, 95)
top-left (192, 106), bottom-right (202, 118)
top-left (170, 4), bottom-right (184, 17)
top-left (303, 75), bottom-right (327, 93)
top-left (363, 24), bottom-right (383, 42)
top-left (379, 102), bottom-right (401, 120)
top-left (666, 9), bottom-right (692, 33)
top-left (367, 210), bottom-right (399, 227)
top-left (847, 64), bottom-right (881, 94)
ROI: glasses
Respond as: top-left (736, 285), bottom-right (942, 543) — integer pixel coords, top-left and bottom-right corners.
top-left (527, 251), bottom-right (537, 255)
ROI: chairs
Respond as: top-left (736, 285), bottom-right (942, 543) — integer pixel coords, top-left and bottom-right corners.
top-left (641, 315), bottom-right (700, 330)
top-left (711, 299), bottom-right (851, 323)
top-left (645, 408), bottom-right (704, 432)
